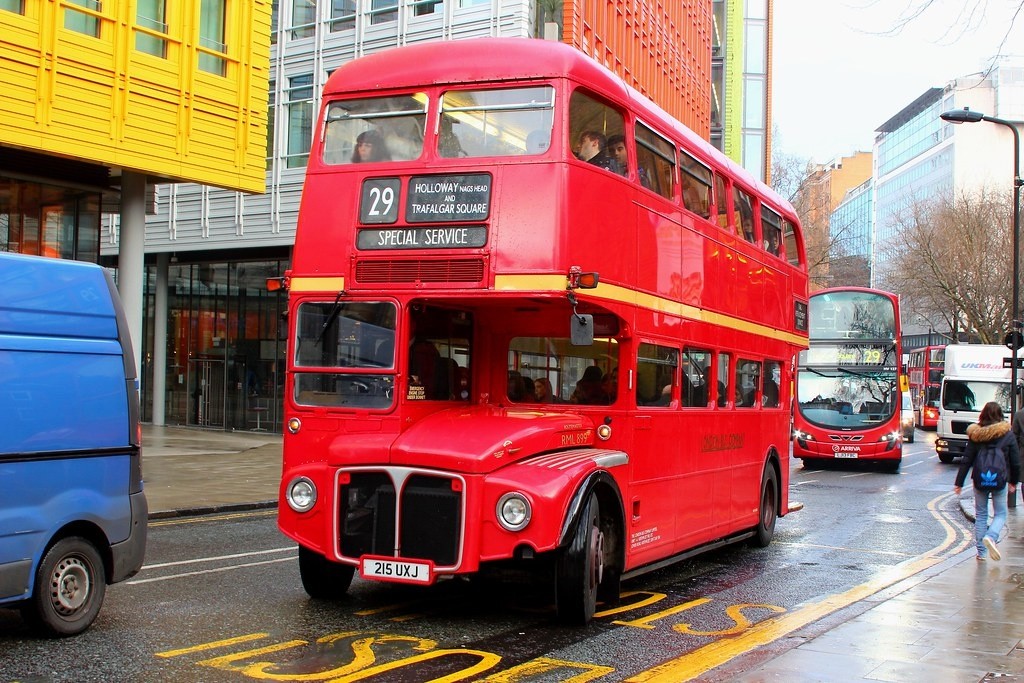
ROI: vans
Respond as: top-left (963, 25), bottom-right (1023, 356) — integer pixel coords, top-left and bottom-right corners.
top-left (0, 249), bottom-right (149, 636)
top-left (900, 386), bottom-right (916, 443)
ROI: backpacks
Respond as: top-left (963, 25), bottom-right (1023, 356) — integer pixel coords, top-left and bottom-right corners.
top-left (974, 431), bottom-right (1008, 492)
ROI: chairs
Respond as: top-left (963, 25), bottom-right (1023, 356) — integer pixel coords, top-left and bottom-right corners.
top-left (828, 402), bottom-right (853, 415)
top-left (441, 356), bottom-right (460, 406)
top-left (508, 370), bottom-right (524, 402)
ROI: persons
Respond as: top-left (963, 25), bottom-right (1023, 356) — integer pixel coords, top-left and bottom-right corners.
top-left (724, 384), bottom-right (744, 407)
top-left (373, 315), bottom-right (441, 399)
top-left (574, 128), bottom-right (650, 188)
top-left (742, 217), bottom-right (755, 234)
top-left (694, 366), bottom-right (725, 408)
top-left (772, 235), bottom-right (788, 261)
top-left (1012, 407), bottom-right (1024, 503)
top-left (508, 370), bottom-right (563, 404)
top-left (570, 366), bottom-right (619, 404)
top-left (352, 131), bottom-right (393, 160)
top-left (954, 403), bottom-right (1021, 561)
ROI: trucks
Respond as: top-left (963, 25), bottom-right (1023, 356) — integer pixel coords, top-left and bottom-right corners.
top-left (928, 343), bottom-right (1024, 463)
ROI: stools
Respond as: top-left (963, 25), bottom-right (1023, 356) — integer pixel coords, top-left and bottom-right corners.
top-left (247, 407), bottom-right (269, 432)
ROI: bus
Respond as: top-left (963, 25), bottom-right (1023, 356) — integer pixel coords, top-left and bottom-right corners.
top-left (790, 286), bottom-right (907, 473)
top-left (266, 36), bottom-right (814, 625)
top-left (908, 344), bottom-right (947, 430)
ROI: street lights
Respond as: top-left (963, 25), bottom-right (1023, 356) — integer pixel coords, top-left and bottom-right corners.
top-left (940, 108), bottom-right (1024, 511)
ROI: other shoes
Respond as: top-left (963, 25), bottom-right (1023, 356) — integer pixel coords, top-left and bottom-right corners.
top-left (983, 535), bottom-right (1001, 560)
top-left (977, 555), bottom-right (985, 559)
